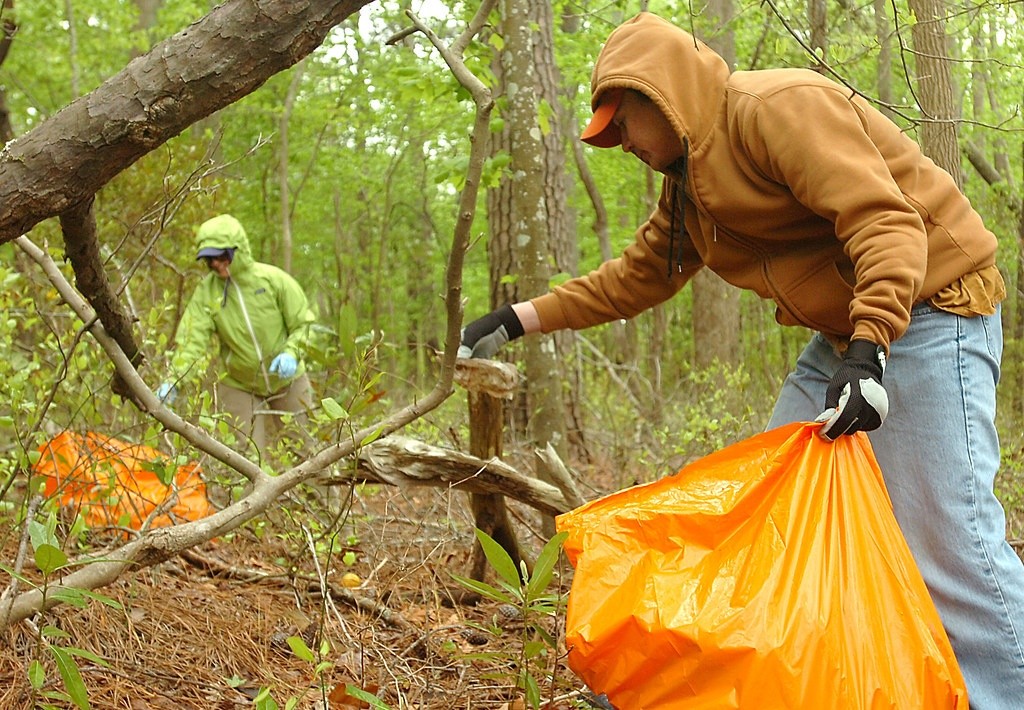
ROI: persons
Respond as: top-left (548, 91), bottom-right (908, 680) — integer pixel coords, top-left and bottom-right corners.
top-left (456, 11), bottom-right (1023, 710)
top-left (156, 214), bottom-right (344, 531)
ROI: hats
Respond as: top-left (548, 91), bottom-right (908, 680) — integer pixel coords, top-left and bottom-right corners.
top-left (196, 247), bottom-right (235, 262)
top-left (581, 87), bottom-right (626, 148)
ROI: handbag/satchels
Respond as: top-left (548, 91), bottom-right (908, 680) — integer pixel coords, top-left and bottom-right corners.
top-left (555, 407), bottom-right (969, 710)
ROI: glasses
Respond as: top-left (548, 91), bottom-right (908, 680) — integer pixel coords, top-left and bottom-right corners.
top-left (203, 250), bottom-right (229, 262)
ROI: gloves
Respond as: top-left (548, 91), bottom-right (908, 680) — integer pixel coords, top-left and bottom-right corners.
top-left (457, 304), bottom-right (525, 360)
top-left (156, 378), bottom-right (176, 406)
top-left (269, 352), bottom-right (297, 378)
top-left (814, 338), bottom-right (889, 442)
